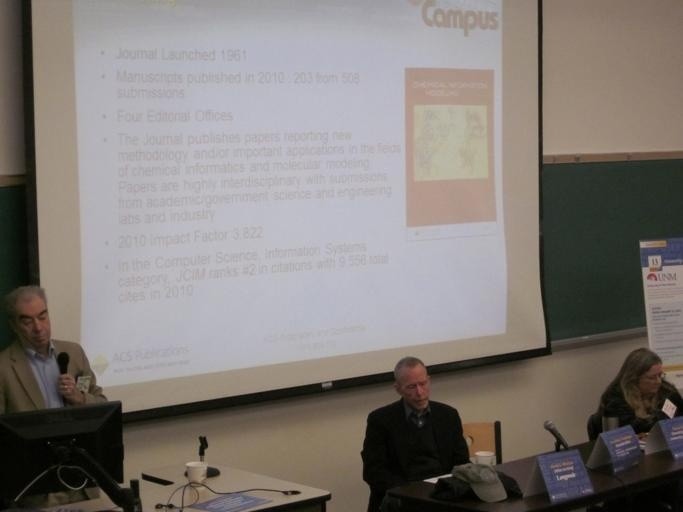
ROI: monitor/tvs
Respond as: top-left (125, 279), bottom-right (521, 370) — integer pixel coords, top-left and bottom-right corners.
top-left (0, 400), bottom-right (136, 512)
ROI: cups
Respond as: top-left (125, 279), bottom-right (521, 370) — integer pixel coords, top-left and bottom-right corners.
top-left (475, 451), bottom-right (494, 465)
top-left (186, 462), bottom-right (207, 487)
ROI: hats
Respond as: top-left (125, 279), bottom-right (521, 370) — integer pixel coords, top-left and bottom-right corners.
top-left (452, 463), bottom-right (508, 503)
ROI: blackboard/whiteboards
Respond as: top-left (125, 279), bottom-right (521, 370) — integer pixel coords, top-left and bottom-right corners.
top-left (0, 151), bottom-right (683, 423)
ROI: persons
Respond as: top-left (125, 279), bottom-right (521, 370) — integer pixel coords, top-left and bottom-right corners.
top-left (0, 286), bottom-right (109, 416)
top-left (593, 347), bottom-right (683, 437)
top-left (361, 356), bottom-right (470, 512)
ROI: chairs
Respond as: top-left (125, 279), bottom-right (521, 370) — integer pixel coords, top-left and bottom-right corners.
top-left (460, 420), bottom-right (503, 469)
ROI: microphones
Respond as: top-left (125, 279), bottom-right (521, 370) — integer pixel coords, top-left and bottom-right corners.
top-left (544, 420), bottom-right (569, 450)
top-left (57, 352), bottom-right (69, 408)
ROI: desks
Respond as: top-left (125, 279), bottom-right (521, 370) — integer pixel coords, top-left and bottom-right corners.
top-left (33, 456), bottom-right (331, 512)
top-left (385, 431), bottom-right (682, 511)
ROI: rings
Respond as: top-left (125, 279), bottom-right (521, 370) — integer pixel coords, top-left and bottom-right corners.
top-left (65, 385), bottom-right (70, 390)
